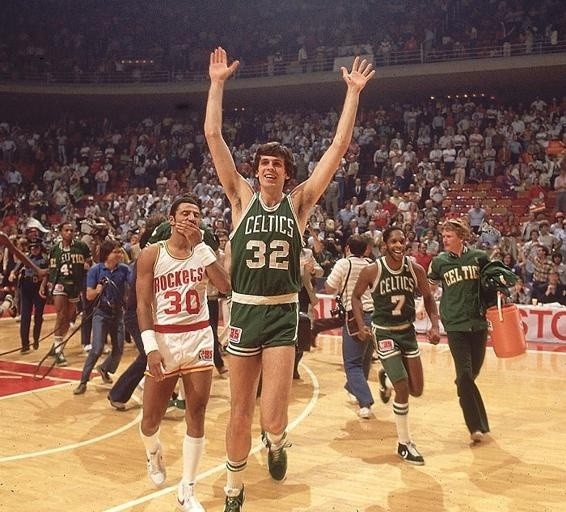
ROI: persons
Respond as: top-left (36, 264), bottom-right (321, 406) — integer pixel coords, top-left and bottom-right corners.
top-left (203, 46), bottom-right (377, 511)
top-left (2, 93), bottom-right (565, 465)
top-left (1, 1), bottom-right (565, 78)
top-left (135, 198), bottom-right (230, 511)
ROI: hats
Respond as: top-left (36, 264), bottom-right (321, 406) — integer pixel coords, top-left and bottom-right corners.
top-left (27, 237), bottom-right (43, 248)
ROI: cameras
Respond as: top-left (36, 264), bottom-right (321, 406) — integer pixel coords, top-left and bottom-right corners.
top-left (330, 304), bottom-right (345, 319)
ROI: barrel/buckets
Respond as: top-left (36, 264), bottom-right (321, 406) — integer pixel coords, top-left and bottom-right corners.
top-left (485, 291), bottom-right (527, 358)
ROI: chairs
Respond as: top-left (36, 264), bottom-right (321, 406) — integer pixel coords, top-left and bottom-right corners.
top-left (439, 177), bottom-right (557, 237)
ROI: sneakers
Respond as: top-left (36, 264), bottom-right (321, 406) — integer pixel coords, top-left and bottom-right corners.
top-left (359, 407), bottom-right (371, 419)
top-left (397, 441), bottom-right (424, 466)
top-left (33, 339), bottom-right (39, 350)
top-left (174, 495), bottom-right (205, 512)
top-left (260, 430), bottom-right (292, 483)
top-left (21, 347), bottom-right (29, 351)
top-left (377, 369), bottom-right (391, 403)
top-left (470, 430), bottom-right (483, 441)
top-left (46, 342), bottom-right (111, 367)
top-left (145, 440), bottom-right (165, 487)
top-left (223, 484), bottom-right (246, 512)
top-left (343, 388), bottom-right (359, 405)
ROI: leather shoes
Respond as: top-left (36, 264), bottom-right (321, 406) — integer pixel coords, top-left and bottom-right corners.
top-left (96, 365), bottom-right (112, 383)
top-left (73, 384), bottom-right (85, 394)
top-left (107, 394), bottom-right (124, 410)
